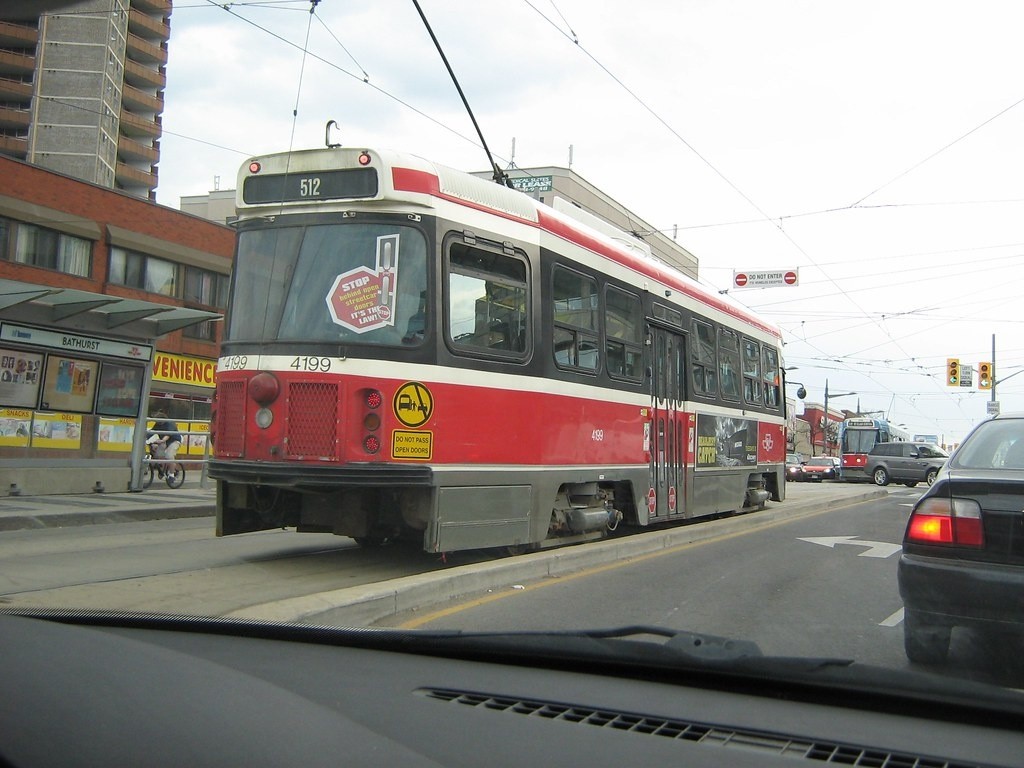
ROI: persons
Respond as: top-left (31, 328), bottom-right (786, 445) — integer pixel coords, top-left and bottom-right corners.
top-left (2, 357), bottom-right (40, 384)
top-left (146, 410), bottom-right (182, 482)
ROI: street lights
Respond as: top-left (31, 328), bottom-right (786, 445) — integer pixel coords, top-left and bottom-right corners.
top-left (857, 397), bottom-right (886, 418)
top-left (823, 378), bottom-right (859, 453)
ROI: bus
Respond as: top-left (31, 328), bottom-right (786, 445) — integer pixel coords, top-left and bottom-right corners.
top-left (840, 416), bottom-right (911, 484)
top-left (205, 136), bottom-right (790, 560)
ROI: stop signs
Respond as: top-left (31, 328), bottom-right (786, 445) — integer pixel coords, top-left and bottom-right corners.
top-left (736, 274), bottom-right (748, 286)
top-left (783, 272), bottom-right (797, 285)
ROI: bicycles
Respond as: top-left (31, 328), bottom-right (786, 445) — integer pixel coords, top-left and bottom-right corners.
top-left (140, 439), bottom-right (187, 489)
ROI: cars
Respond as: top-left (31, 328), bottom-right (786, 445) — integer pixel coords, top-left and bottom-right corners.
top-left (785, 453), bottom-right (807, 483)
top-left (897, 417), bottom-right (1023, 674)
top-left (799, 457), bottom-right (843, 481)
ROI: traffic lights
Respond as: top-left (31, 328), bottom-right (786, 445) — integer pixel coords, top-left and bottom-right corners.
top-left (977, 362), bottom-right (991, 390)
top-left (946, 358), bottom-right (960, 386)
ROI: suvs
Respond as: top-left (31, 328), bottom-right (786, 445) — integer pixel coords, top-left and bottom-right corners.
top-left (862, 441), bottom-right (951, 487)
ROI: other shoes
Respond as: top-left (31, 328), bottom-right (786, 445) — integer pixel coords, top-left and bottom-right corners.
top-left (158, 472), bottom-right (163, 479)
top-left (169, 476), bottom-right (175, 482)
